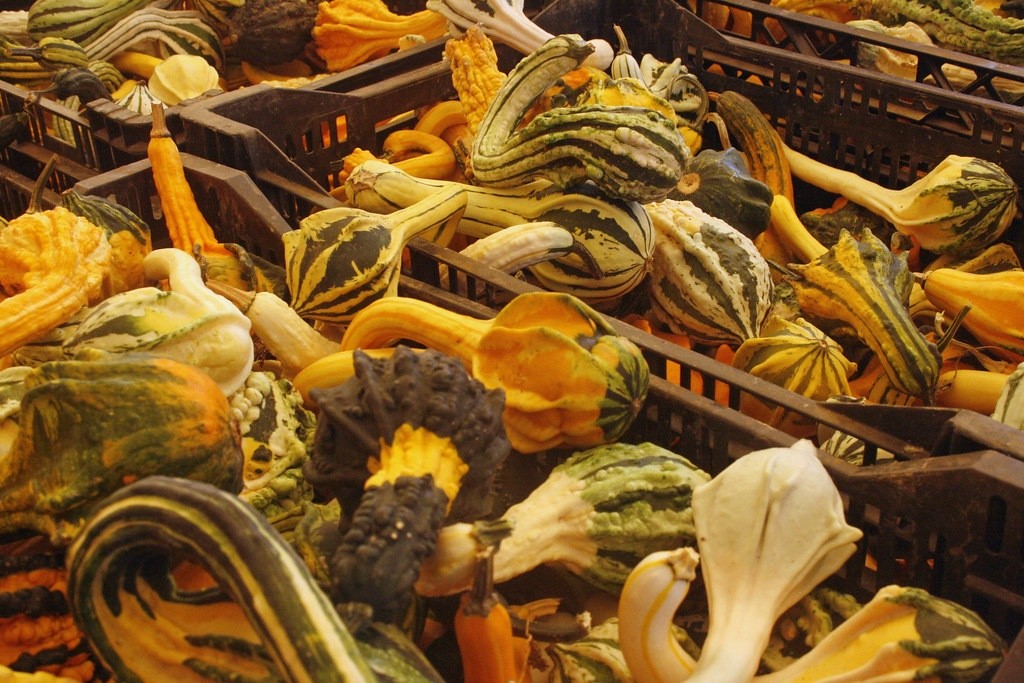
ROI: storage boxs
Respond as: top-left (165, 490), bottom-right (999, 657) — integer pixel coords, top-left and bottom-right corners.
top-left (1, 0), bottom-right (1024, 682)
